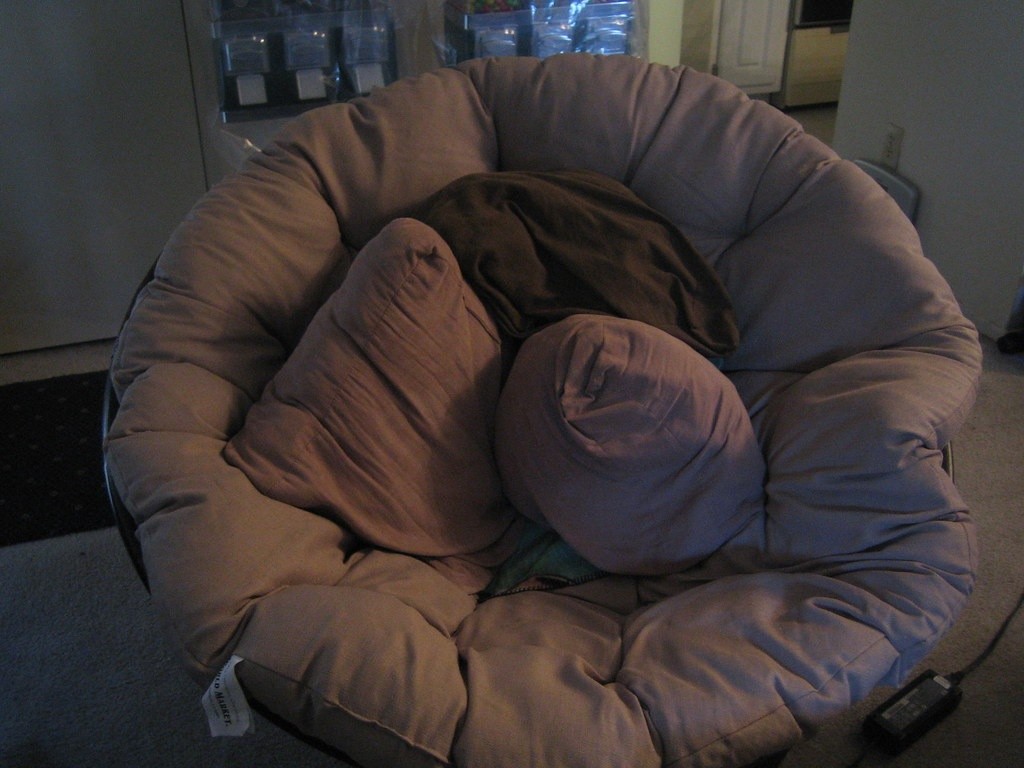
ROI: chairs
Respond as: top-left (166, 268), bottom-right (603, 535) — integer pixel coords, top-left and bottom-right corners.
top-left (101, 52), bottom-right (987, 768)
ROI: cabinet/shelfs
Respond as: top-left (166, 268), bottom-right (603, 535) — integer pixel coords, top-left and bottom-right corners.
top-left (680, 0), bottom-right (851, 111)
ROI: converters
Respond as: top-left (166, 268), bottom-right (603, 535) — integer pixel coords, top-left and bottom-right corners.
top-left (865, 668), bottom-right (963, 758)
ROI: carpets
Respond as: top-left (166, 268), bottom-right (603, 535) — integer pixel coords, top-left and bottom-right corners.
top-left (0, 370), bottom-right (114, 550)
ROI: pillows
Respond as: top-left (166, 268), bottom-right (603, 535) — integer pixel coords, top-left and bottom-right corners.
top-left (413, 167), bottom-right (740, 361)
top-left (495, 313), bottom-right (768, 581)
top-left (225, 215), bottom-right (530, 594)
